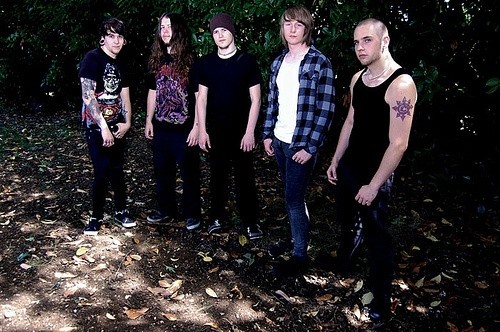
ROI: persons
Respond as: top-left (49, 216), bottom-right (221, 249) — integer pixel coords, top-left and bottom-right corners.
top-left (78, 17), bottom-right (137, 235)
top-left (196, 13), bottom-right (264, 241)
top-left (326, 17), bottom-right (419, 324)
top-left (263, 7), bottom-right (336, 277)
top-left (144, 11), bottom-right (202, 230)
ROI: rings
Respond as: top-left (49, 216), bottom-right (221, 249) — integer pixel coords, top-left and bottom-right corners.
top-left (366, 202), bottom-right (369, 204)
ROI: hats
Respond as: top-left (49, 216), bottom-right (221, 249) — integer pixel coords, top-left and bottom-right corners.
top-left (210, 13), bottom-right (236, 37)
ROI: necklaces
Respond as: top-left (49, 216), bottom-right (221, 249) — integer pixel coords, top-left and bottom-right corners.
top-left (367, 61), bottom-right (391, 80)
top-left (218, 48), bottom-right (237, 56)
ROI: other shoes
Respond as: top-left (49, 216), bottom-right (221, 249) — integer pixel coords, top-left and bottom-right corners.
top-left (185, 217), bottom-right (200, 230)
top-left (247, 224), bottom-right (264, 239)
top-left (207, 221), bottom-right (231, 234)
top-left (114, 209), bottom-right (137, 228)
top-left (369, 300), bottom-right (387, 320)
top-left (83, 215), bottom-right (103, 234)
top-left (277, 256), bottom-right (308, 271)
top-left (146, 210), bottom-right (170, 222)
top-left (331, 256), bottom-right (361, 268)
